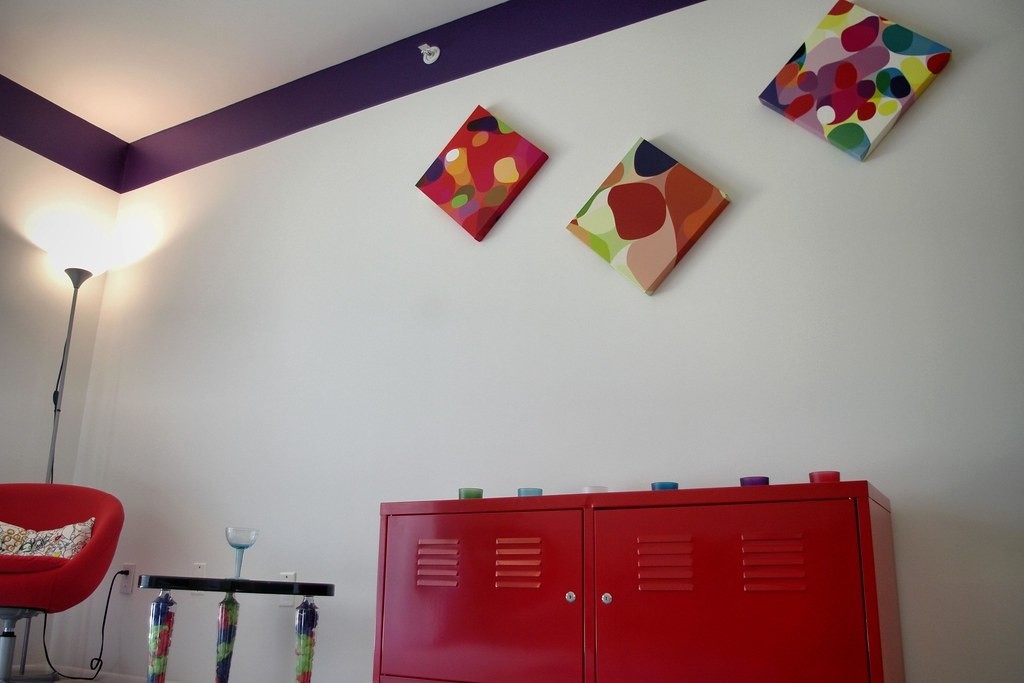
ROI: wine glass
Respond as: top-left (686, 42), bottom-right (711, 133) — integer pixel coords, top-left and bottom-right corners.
top-left (226, 527), bottom-right (260, 580)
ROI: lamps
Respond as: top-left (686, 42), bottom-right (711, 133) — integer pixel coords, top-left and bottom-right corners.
top-left (16, 214), bottom-right (125, 683)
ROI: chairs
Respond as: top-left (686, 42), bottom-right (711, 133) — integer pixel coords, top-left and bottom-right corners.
top-left (0, 481), bottom-right (125, 683)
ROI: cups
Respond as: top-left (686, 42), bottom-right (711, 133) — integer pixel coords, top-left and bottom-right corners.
top-left (740, 476), bottom-right (769, 486)
top-left (809, 471), bottom-right (840, 483)
top-left (650, 481), bottom-right (678, 491)
top-left (459, 488), bottom-right (483, 500)
top-left (517, 488), bottom-right (542, 497)
top-left (585, 486), bottom-right (608, 493)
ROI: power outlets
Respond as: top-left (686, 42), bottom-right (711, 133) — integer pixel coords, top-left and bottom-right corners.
top-left (119, 563), bottom-right (136, 594)
top-left (190, 563), bottom-right (207, 596)
top-left (278, 573), bottom-right (296, 607)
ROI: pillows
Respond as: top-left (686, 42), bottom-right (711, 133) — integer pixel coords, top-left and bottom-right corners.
top-left (0, 517), bottom-right (96, 560)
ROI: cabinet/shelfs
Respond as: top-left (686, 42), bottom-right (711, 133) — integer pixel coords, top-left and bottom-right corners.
top-left (371, 480), bottom-right (907, 683)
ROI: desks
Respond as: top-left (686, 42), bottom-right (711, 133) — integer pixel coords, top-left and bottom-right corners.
top-left (136, 572), bottom-right (334, 683)
